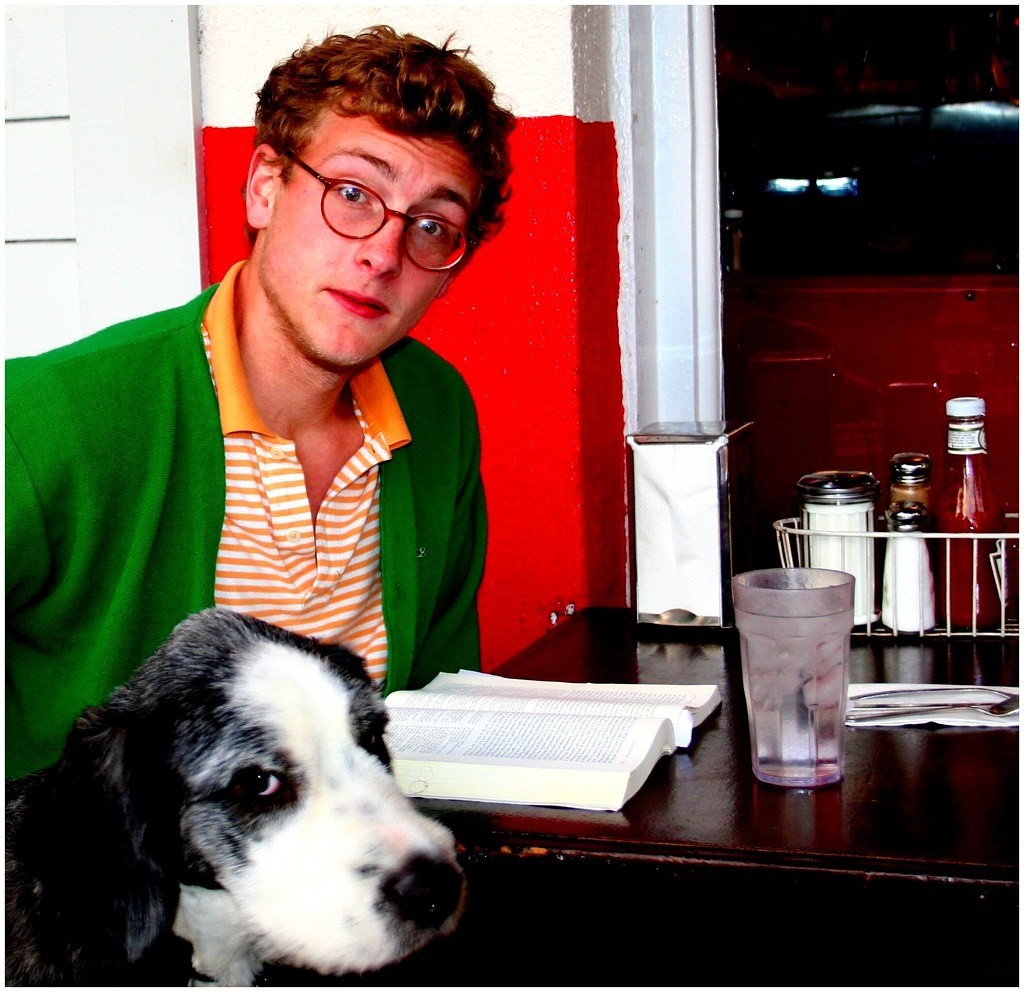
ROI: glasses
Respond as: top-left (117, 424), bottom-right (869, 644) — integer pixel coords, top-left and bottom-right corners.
top-left (284, 148), bottom-right (479, 272)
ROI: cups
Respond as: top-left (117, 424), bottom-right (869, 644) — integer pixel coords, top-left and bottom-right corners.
top-left (732, 567), bottom-right (855, 787)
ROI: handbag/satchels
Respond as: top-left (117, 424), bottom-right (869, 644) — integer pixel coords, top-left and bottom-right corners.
top-left (843, 681), bottom-right (1019, 727)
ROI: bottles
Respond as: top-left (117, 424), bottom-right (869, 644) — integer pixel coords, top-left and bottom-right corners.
top-left (940, 398), bottom-right (1009, 628)
top-left (891, 453), bottom-right (931, 508)
top-left (798, 470), bottom-right (877, 632)
top-left (881, 500), bottom-right (935, 632)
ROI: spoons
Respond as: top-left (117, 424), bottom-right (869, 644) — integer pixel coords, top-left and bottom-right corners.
top-left (844, 694), bottom-right (1020, 722)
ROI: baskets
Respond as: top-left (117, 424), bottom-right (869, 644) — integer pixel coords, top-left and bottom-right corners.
top-left (774, 514), bottom-right (1019, 637)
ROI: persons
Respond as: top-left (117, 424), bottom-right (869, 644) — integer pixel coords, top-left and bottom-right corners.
top-left (6, 24), bottom-right (521, 787)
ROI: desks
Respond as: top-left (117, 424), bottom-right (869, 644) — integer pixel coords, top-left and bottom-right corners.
top-left (407, 603), bottom-right (1019, 987)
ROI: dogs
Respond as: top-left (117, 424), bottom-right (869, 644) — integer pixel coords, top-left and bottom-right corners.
top-left (5, 605), bottom-right (466, 987)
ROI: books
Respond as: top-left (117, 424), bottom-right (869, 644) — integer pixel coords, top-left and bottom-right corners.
top-left (378, 668), bottom-right (723, 813)
